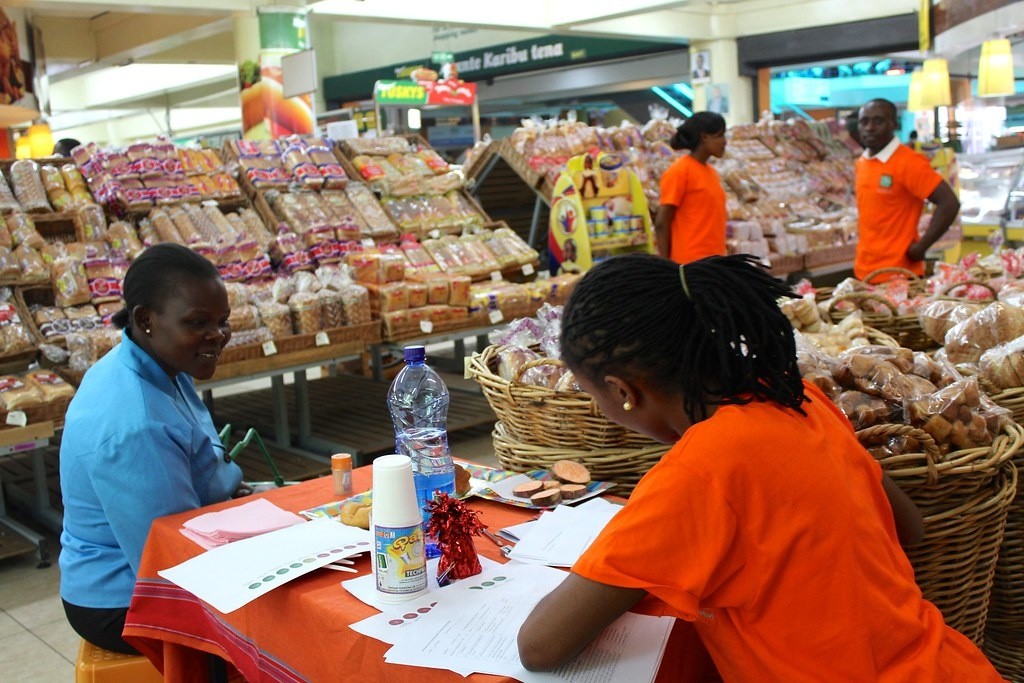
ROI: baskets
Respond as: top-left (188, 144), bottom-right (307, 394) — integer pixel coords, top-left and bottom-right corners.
top-left (464, 267), bottom-right (1024, 683)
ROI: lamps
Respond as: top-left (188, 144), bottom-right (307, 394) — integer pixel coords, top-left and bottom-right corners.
top-left (907, 66), bottom-right (935, 113)
top-left (977, 1), bottom-right (1015, 98)
top-left (921, 0), bottom-right (953, 108)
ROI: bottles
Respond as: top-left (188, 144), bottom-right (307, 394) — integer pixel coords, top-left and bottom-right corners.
top-left (330, 453), bottom-right (353, 495)
top-left (384, 345), bottom-right (459, 558)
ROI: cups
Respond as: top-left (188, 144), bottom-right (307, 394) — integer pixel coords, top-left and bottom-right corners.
top-left (369, 455), bottom-right (428, 605)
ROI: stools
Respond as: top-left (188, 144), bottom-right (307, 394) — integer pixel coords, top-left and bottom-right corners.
top-left (76, 638), bottom-right (165, 683)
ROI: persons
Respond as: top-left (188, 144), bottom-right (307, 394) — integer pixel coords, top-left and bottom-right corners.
top-left (56, 241), bottom-right (244, 655)
top-left (514, 249), bottom-right (1006, 682)
top-left (852, 97), bottom-right (963, 285)
top-left (654, 111), bottom-right (729, 264)
top-left (51, 138), bottom-right (81, 156)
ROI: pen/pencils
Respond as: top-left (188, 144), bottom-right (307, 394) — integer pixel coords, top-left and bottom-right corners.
top-left (481, 528), bottom-right (504, 547)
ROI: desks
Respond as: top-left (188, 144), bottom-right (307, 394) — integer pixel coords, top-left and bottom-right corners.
top-left (121, 453), bottom-right (711, 683)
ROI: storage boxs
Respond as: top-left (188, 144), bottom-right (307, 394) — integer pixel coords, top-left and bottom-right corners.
top-left (0, 158), bottom-right (76, 235)
top-left (192, 317), bottom-right (383, 365)
top-left (757, 250), bottom-right (804, 274)
top-left (500, 136), bottom-right (546, 190)
top-left (96, 146), bottom-right (248, 225)
top-left (467, 139), bottom-right (502, 180)
top-left (539, 177), bottom-right (554, 201)
top-left (321, 347), bottom-right (408, 383)
top-left (51, 364), bottom-right (85, 389)
top-left (0, 280), bottom-right (41, 376)
top-left (122, 196), bottom-right (265, 246)
top-left (802, 234), bottom-right (858, 267)
top-left (219, 133), bottom-right (539, 282)
top-left (0, 395), bottom-right (76, 429)
top-left (0, 419), bottom-right (55, 457)
top-left (19, 284), bottom-right (72, 371)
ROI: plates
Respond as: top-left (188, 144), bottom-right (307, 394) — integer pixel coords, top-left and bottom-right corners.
top-left (298, 490), bottom-right (372, 522)
top-left (453, 460), bottom-right (515, 502)
top-left (476, 472), bottom-right (617, 510)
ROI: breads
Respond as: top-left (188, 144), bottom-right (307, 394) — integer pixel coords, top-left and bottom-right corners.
top-left (509, 114), bottom-right (962, 259)
top-left (0, 132), bottom-right (588, 431)
top-left (495, 246), bottom-right (1024, 461)
top-left (340, 498), bottom-right (371, 530)
top-left (453, 463), bottom-right (471, 498)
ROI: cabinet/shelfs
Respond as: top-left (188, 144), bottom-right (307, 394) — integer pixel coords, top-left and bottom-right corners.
top-left (0, 421), bottom-right (67, 571)
top-left (193, 337), bottom-right (499, 482)
top-left (369, 322), bottom-right (508, 400)
